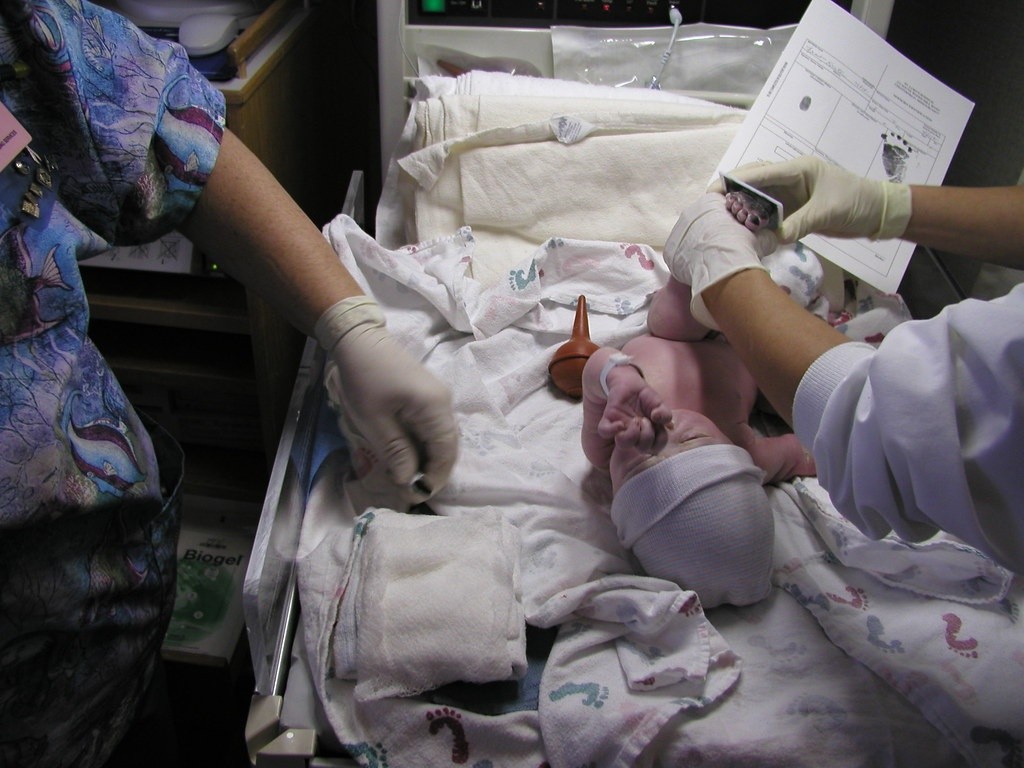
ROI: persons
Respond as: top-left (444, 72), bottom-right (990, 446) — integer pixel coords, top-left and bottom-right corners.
top-left (663, 157), bottom-right (1023, 579)
top-left (0, 0), bottom-right (462, 766)
top-left (581, 193), bottom-right (816, 610)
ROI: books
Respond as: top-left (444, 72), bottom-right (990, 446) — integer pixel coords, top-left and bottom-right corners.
top-left (161, 495), bottom-right (261, 665)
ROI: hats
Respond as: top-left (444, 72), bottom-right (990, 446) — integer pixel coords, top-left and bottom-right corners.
top-left (610, 444), bottom-right (775, 607)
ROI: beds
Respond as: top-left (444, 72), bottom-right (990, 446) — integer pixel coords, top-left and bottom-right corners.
top-left (244, 0), bottom-right (991, 768)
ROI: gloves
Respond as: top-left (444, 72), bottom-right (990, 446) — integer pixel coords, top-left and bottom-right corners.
top-left (707, 155), bottom-right (913, 241)
top-left (662, 193), bottom-right (777, 331)
top-left (314, 295), bottom-right (457, 504)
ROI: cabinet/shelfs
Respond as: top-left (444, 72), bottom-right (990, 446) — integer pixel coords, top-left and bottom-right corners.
top-left (76, 7), bottom-right (336, 505)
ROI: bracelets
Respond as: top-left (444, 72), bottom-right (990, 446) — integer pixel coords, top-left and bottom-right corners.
top-left (600, 354), bottom-right (646, 396)
